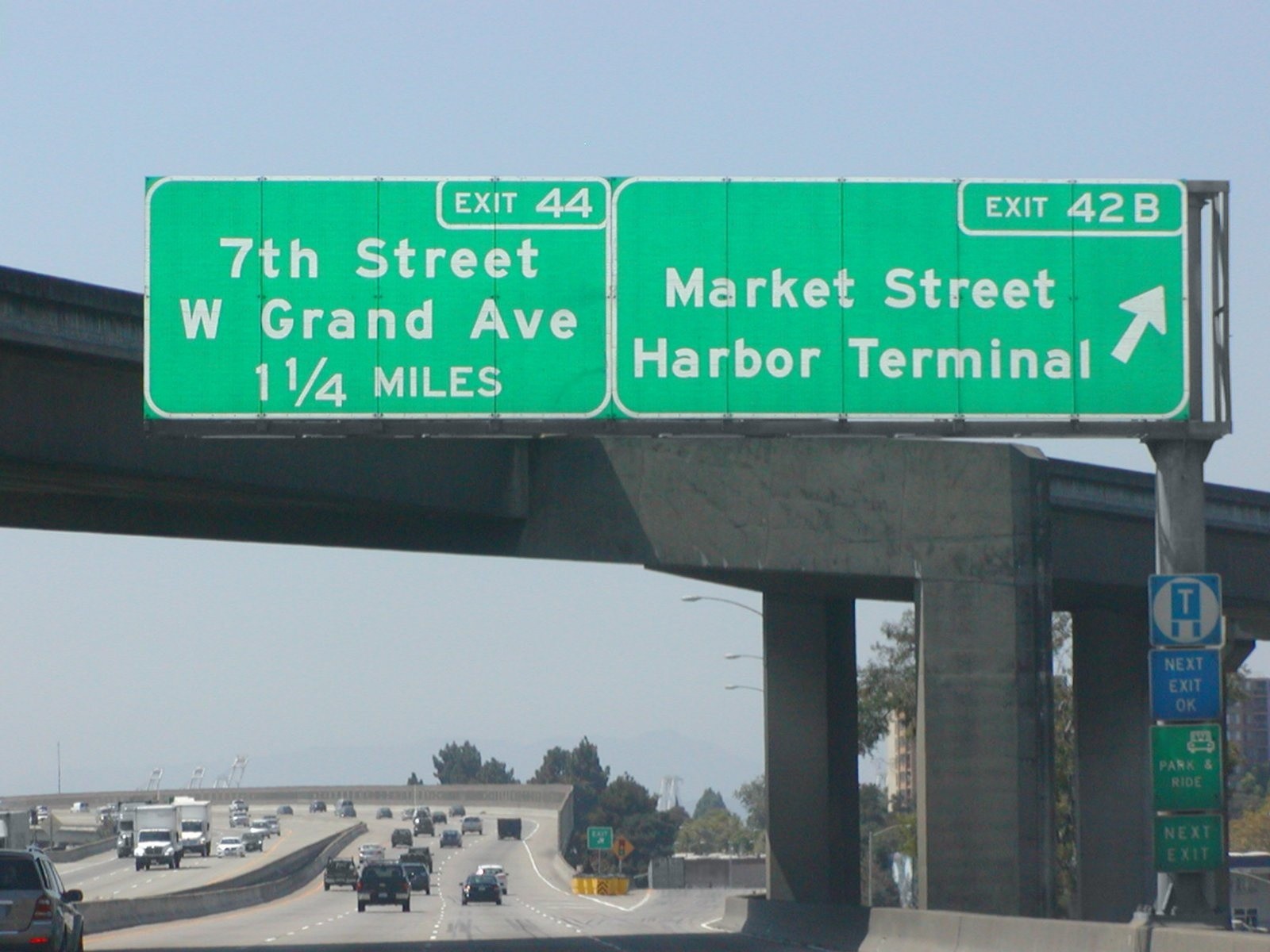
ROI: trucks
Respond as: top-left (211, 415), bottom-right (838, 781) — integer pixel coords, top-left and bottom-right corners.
top-left (129, 804), bottom-right (185, 871)
top-left (0, 811), bottom-right (38, 852)
top-left (70, 802), bottom-right (90, 813)
top-left (497, 817), bottom-right (522, 841)
top-left (174, 801), bottom-right (213, 854)
top-left (116, 801), bottom-right (147, 859)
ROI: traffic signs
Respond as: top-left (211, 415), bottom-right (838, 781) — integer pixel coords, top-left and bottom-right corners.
top-left (588, 826), bottom-right (614, 851)
top-left (604, 175), bottom-right (1190, 436)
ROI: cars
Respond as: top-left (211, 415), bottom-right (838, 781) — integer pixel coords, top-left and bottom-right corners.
top-left (277, 806), bottom-right (295, 815)
top-left (96, 805), bottom-right (120, 828)
top-left (440, 830), bottom-right (463, 849)
top-left (29, 805), bottom-right (53, 825)
top-left (334, 797), bottom-right (360, 817)
top-left (241, 831), bottom-right (265, 851)
top-left (462, 818), bottom-right (483, 834)
top-left (309, 801), bottom-right (327, 813)
top-left (215, 837), bottom-right (245, 857)
top-left (476, 865), bottom-right (512, 890)
top-left (459, 874), bottom-right (504, 907)
top-left (358, 802), bottom-right (465, 873)
top-left (227, 799), bottom-right (279, 838)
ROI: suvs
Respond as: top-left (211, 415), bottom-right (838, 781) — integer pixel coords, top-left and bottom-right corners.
top-left (355, 860), bottom-right (414, 912)
top-left (399, 863), bottom-right (432, 895)
top-left (324, 858), bottom-right (358, 890)
top-left (0, 847), bottom-right (86, 952)
